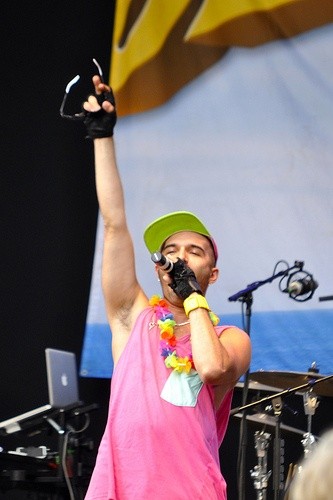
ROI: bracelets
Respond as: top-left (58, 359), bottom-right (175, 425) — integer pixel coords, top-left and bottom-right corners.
top-left (183, 295), bottom-right (210, 316)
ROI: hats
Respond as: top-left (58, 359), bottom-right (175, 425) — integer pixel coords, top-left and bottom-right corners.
top-left (143, 211), bottom-right (218, 261)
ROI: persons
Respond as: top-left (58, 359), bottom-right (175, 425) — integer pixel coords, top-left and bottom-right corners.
top-left (84, 75), bottom-right (252, 500)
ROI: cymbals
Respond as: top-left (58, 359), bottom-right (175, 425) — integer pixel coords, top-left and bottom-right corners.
top-left (232, 412), bottom-right (318, 441)
top-left (234, 382), bottom-right (304, 395)
top-left (249, 371), bottom-right (333, 396)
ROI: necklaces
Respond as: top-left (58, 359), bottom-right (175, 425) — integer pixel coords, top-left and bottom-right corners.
top-left (148, 295), bottom-right (220, 373)
top-left (175, 321), bottom-right (190, 327)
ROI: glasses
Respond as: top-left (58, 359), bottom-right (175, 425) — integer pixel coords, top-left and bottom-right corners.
top-left (60, 59), bottom-right (103, 120)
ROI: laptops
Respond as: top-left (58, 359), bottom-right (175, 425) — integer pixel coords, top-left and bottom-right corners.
top-left (0, 347), bottom-right (79, 429)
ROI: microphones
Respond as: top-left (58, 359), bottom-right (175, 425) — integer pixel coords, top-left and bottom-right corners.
top-left (287, 278), bottom-right (318, 295)
top-left (151, 251), bottom-right (176, 284)
